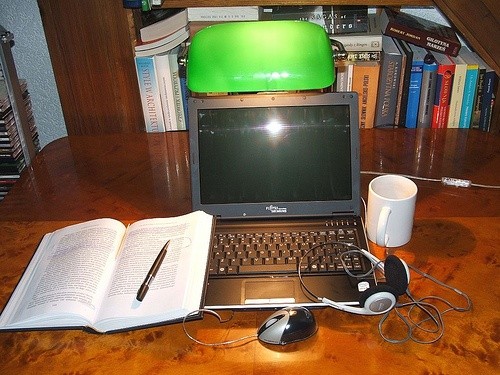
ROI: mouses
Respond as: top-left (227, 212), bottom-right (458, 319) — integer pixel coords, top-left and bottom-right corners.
top-left (257, 306), bottom-right (315, 345)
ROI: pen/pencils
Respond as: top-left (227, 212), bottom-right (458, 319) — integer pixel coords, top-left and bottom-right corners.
top-left (136, 240), bottom-right (171, 302)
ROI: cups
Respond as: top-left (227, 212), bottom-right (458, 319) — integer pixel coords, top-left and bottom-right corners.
top-left (366, 174), bottom-right (419, 248)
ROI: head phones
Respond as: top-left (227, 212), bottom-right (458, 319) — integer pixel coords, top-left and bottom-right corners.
top-left (297, 238), bottom-right (411, 316)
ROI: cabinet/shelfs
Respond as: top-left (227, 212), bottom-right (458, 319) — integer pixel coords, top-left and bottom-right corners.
top-left (0, 32), bottom-right (39, 203)
top-left (37, 0), bottom-right (500, 133)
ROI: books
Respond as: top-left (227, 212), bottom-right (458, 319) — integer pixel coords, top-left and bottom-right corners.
top-left (0, 63), bottom-right (41, 200)
top-left (134, 6), bottom-right (495, 133)
top-left (0, 209), bottom-right (217, 335)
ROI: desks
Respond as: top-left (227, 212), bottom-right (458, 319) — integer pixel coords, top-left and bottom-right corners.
top-left (0, 129), bottom-right (497, 374)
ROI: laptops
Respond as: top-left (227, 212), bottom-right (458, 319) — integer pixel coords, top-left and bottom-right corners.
top-left (188, 92), bottom-right (377, 312)
